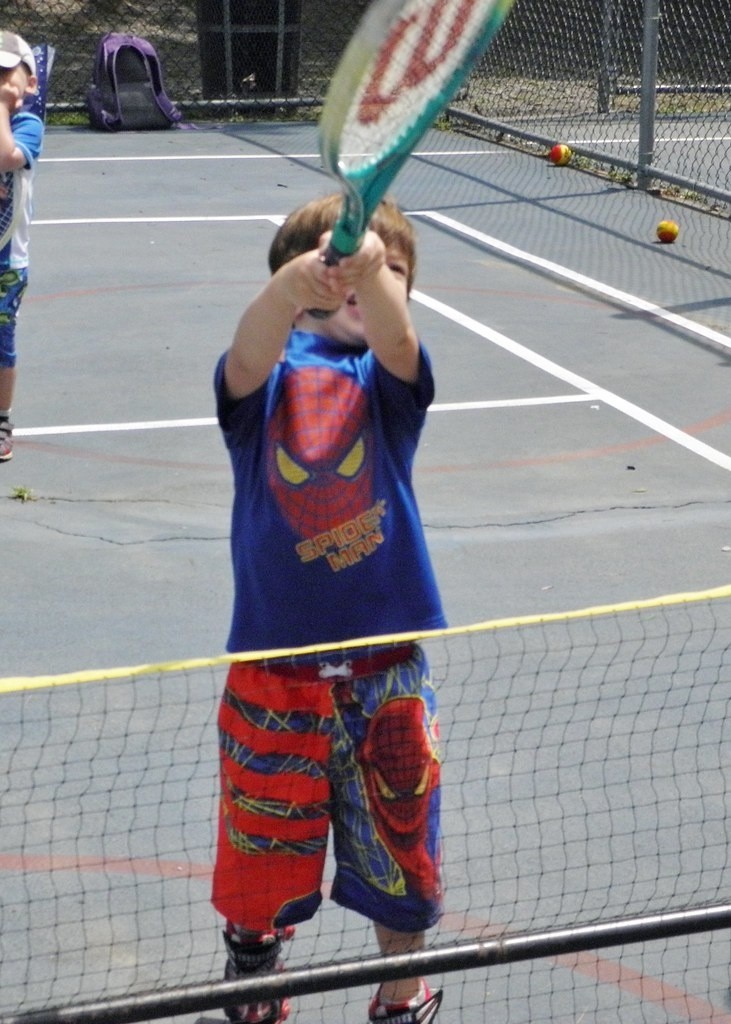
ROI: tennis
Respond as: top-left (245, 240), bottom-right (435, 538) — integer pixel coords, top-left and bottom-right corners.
top-left (656, 219), bottom-right (680, 242)
top-left (550, 144), bottom-right (572, 166)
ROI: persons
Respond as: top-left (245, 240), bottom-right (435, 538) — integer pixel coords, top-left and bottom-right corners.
top-left (214, 192), bottom-right (449, 1024)
top-left (0, 27), bottom-right (44, 460)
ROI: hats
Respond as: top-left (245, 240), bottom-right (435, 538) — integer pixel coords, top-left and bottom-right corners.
top-left (0, 31), bottom-right (36, 76)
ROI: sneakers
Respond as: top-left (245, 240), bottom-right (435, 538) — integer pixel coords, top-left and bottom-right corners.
top-left (0, 421), bottom-right (15, 461)
top-left (366, 981), bottom-right (443, 1024)
top-left (222, 918), bottom-right (295, 1024)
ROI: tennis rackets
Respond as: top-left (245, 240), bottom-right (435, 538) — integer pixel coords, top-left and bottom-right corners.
top-left (307, 0), bottom-right (517, 324)
